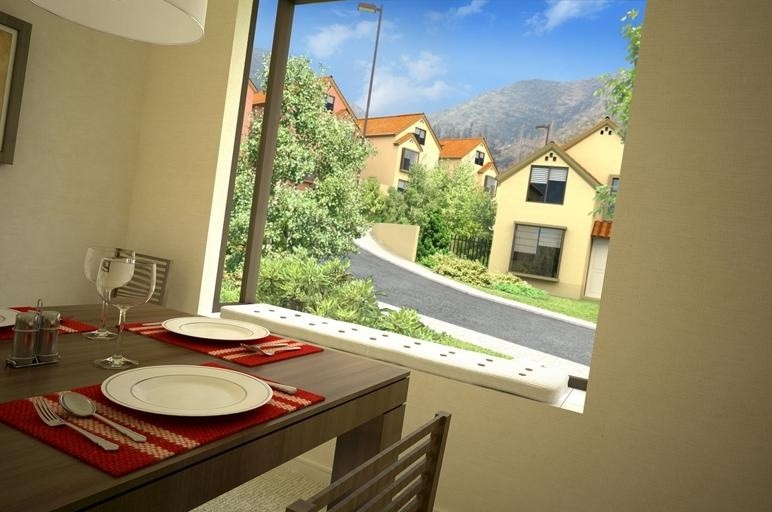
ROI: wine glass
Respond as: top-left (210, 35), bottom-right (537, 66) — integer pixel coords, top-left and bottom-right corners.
top-left (94, 259), bottom-right (157, 369)
top-left (81, 246), bottom-right (134, 342)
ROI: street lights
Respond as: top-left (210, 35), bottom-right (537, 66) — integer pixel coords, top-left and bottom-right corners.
top-left (357, 3), bottom-right (384, 183)
top-left (535, 123), bottom-right (551, 146)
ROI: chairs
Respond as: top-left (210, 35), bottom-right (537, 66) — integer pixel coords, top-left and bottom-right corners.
top-left (283, 409), bottom-right (452, 511)
top-left (110, 247), bottom-right (171, 306)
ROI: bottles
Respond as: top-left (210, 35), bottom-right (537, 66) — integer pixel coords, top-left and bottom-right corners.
top-left (9, 311), bottom-right (62, 370)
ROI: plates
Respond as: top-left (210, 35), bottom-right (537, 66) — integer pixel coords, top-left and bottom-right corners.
top-left (0, 305), bottom-right (22, 329)
top-left (100, 364), bottom-right (274, 417)
top-left (163, 317), bottom-right (271, 340)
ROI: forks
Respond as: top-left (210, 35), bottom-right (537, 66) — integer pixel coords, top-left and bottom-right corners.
top-left (30, 398), bottom-right (120, 451)
top-left (255, 344), bottom-right (302, 357)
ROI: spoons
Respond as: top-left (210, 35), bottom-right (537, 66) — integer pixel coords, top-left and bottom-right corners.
top-left (240, 344), bottom-right (288, 351)
top-left (60, 391), bottom-right (148, 443)
